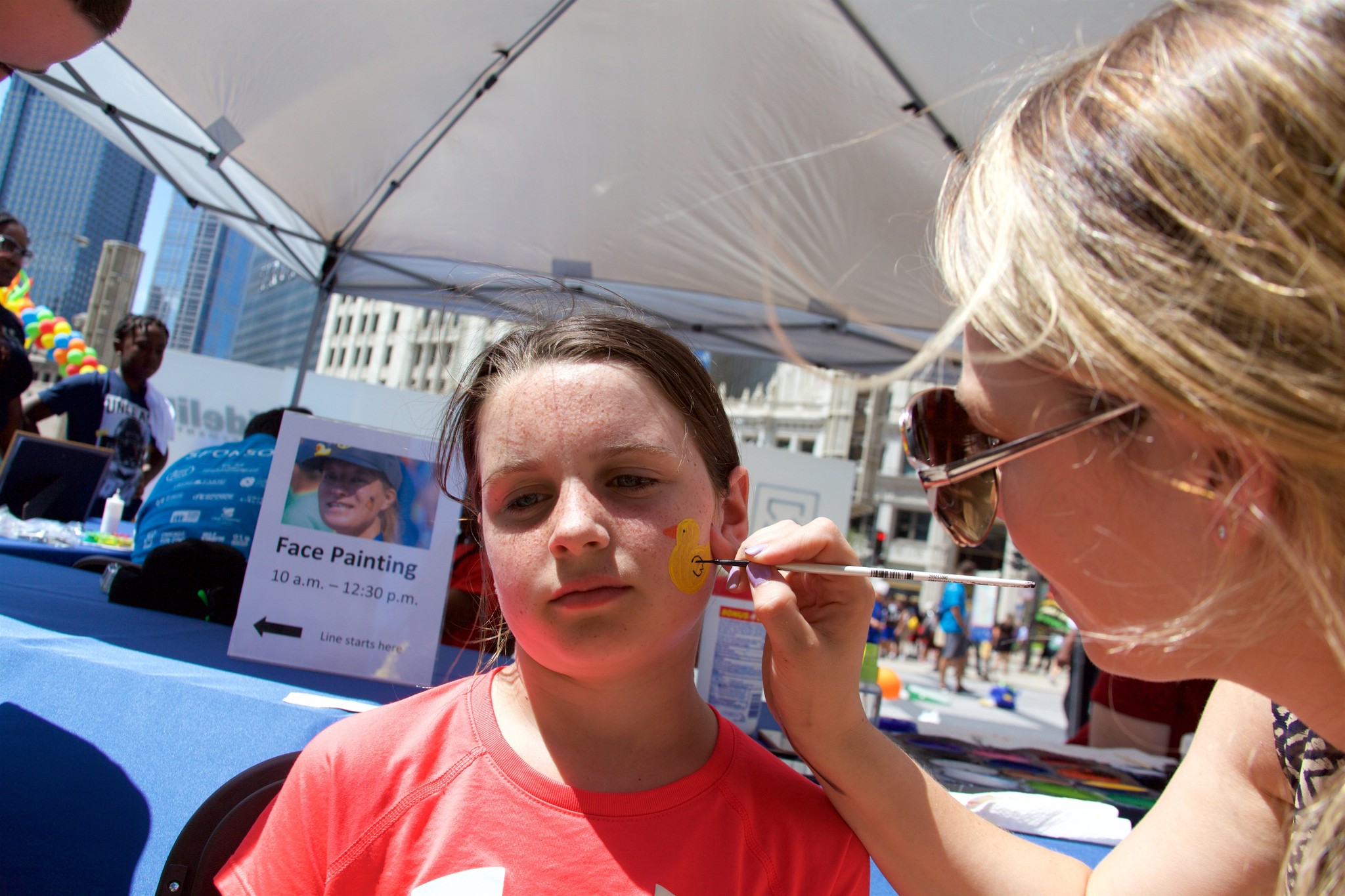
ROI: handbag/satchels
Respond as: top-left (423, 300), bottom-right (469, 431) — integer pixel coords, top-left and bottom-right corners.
top-left (930, 605), bottom-right (944, 622)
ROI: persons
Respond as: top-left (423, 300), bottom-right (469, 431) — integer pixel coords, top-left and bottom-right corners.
top-left (1, 0), bottom-right (133, 84)
top-left (730, 0), bottom-right (1345, 896)
top-left (23, 312), bottom-right (176, 521)
top-left (0, 209), bottom-right (35, 458)
top-left (284, 438), bottom-right (403, 543)
top-left (439, 501), bottom-right (517, 657)
top-left (205, 311), bottom-right (870, 894)
top-left (131, 406), bottom-right (311, 567)
top-left (858, 558), bottom-right (1053, 692)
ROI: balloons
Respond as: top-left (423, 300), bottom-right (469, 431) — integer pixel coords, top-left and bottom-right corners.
top-left (0, 269), bottom-right (106, 378)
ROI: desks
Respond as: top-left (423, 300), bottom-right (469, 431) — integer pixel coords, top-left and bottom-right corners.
top-left (0, 511), bottom-right (1110, 895)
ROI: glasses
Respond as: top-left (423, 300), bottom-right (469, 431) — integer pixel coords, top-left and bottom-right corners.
top-left (0, 234), bottom-right (34, 264)
top-left (902, 387), bottom-right (1143, 548)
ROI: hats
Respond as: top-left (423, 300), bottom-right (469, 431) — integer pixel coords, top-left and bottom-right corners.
top-left (301, 445), bottom-right (402, 493)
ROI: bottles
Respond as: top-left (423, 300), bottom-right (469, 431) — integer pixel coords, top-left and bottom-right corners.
top-left (99, 488), bottom-right (125, 534)
